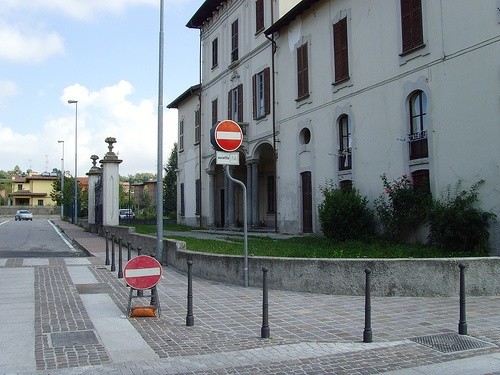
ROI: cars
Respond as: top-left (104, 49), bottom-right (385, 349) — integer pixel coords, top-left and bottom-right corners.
top-left (14, 209), bottom-right (32, 221)
top-left (119, 208), bottom-right (135, 220)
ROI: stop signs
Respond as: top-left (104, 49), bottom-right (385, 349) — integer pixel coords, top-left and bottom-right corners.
top-left (209, 121), bottom-right (244, 153)
top-left (123, 256), bottom-right (164, 293)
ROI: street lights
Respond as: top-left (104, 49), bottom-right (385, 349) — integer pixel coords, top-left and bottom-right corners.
top-left (59, 141), bottom-right (64, 220)
top-left (69, 100), bottom-right (78, 226)
